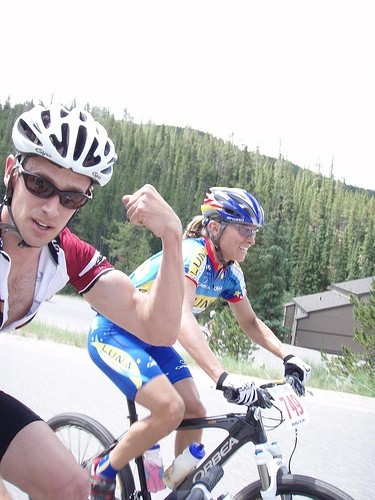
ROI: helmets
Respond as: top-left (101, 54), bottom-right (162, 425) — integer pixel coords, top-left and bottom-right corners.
top-left (12, 103), bottom-right (117, 186)
top-left (201, 186), bottom-right (265, 228)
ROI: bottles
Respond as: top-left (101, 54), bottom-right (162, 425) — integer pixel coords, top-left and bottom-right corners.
top-left (161, 443), bottom-right (206, 490)
top-left (144, 443), bottom-right (166, 492)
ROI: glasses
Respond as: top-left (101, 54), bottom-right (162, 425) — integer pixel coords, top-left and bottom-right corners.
top-left (16, 159), bottom-right (93, 209)
top-left (219, 220), bottom-right (257, 238)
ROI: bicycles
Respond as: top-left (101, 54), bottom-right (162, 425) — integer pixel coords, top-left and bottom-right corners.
top-left (24, 368), bottom-right (357, 500)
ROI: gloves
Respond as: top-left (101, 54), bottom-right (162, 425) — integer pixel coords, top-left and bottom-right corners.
top-left (218, 372), bottom-right (258, 407)
top-left (284, 354), bottom-right (312, 387)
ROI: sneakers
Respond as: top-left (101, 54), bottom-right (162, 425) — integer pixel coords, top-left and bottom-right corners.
top-left (86, 457), bottom-right (117, 500)
top-left (162, 464), bottom-right (178, 491)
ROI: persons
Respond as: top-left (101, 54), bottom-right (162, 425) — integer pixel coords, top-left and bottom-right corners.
top-left (83, 187), bottom-right (311, 500)
top-left (0, 105), bottom-right (184, 500)
